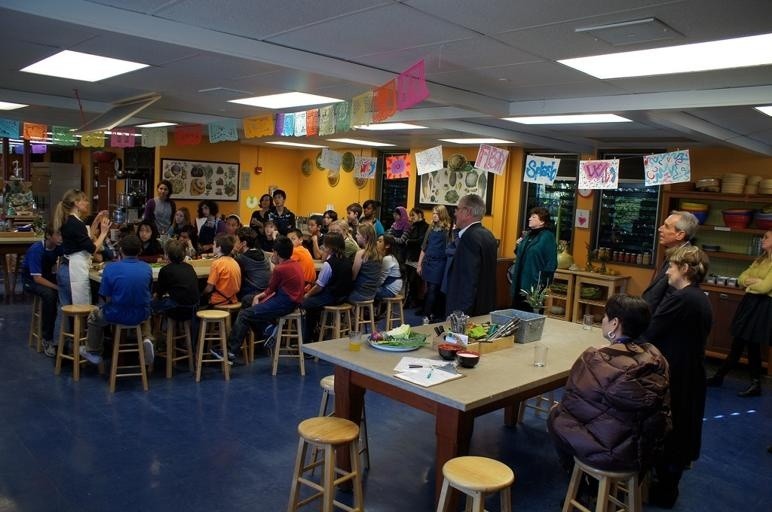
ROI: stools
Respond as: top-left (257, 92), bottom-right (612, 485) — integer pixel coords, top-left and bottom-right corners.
top-left (196, 310), bottom-right (230, 382)
top-left (309, 375), bottom-right (369, 473)
top-left (270, 306), bottom-right (308, 377)
top-left (561, 454), bottom-right (643, 512)
top-left (108, 322), bottom-right (149, 392)
top-left (312, 306), bottom-right (352, 363)
top-left (54, 304), bottom-right (97, 382)
top-left (351, 300), bottom-right (377, 336)
top-left (153, 313), bottom-right (194, 379)
top-left (205, 302), bottom-right (249, 367)
top-left (377, 295), bottom-right (405, 331)
top-left (28, 295), bottom-right (44, 354)
top-left (249, 328), bottom-right (273, 362)
top-left (435, 455), bottom-right (514, 512)
top-left (518, 390), bottom-right (554, 431)
top-left (287, 416), bottom-right (363, 512)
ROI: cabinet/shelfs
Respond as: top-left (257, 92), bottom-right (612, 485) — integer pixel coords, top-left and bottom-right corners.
top-left (574, 272), bottom-right (630, 327)
top-left (661, 189), bottom-right (772, 376)
top-left (544, 268), bottom-right (573, 321)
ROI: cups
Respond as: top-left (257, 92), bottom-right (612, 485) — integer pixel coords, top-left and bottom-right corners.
top-left (349, 330), bottom-right (364, 353)
top-left (582, 314), bottom-right (594, 333)
top-left (533, 345), bottom-right (549, 368)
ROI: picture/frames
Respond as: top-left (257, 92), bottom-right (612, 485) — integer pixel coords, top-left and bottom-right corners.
top-left (161, 158), bottom-right (240, 203)
top-left (415, 160), bottom-right (494, 216)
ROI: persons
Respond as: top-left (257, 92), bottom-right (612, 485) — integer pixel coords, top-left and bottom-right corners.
top-left (542, 292), bottom-right (673, 491)
top-left (209, 190), bottom-right (403, 364)
top-left (510, 207), bottom-right (558, 314)
top-left (23, 182), bottom-right (242, 365)
top-left (637, 209), bottom-right (704, 319)
top-left (641, 243), bottom-right (714, 510)
top-left (388, 194), bottom-right (496, 328)
top-left (705, 229), bottom-right (771, 396)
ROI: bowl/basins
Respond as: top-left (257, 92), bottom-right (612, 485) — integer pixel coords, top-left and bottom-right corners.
top-left (437, 344), bottom-right (463, 360)
top-left (721, 207), bottom-right (771, 231)
top-left (456, 350), bottom-right (480, 367)
top-left (677, 201), bottom-right (709, 224)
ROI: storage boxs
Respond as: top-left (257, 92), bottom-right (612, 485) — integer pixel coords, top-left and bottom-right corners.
top-left (490, 309), bottom-right (546, 344)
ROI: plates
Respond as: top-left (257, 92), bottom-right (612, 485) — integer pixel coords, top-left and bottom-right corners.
top-left (669, 174), bottom-right (772, 194)
top-left (368, 339), bottom-right (420, 353)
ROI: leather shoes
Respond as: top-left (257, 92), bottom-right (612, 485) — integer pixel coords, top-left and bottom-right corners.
top-left (705, 374), bottom-right (724, 387)
top-left (737, 378), bottom-right (762, 397)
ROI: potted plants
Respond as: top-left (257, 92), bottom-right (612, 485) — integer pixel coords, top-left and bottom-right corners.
top-left (555, 239), bottom-right (573, 268)
top-left (520, 270), bottom-right (551, 314)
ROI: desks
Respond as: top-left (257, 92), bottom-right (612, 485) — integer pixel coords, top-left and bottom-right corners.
top-left (89, 257), bottom-right (325, 342)
top-left (0, 232), bottom-right (41, 303)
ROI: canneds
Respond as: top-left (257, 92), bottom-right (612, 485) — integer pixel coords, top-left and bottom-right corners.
top-left (598, 247), bottom-right (643, 264)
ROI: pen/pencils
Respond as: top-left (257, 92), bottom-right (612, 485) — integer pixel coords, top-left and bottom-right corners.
top-left (486, 316), bottom-right (521, 341)
top-left (427, 368), bottom-right (433, 379)
top-left (449, 310), bottom-right (467, 334)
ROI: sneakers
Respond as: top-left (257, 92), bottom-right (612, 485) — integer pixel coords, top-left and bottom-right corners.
top-left (79, 345), bottom-right (103, 365)
top-left (209, 348), bottom-right (236, 366)
top-left (264, 324), bottom-right (278, 349)
top-left (41, 338), bottom-right (57, 358)
top-left (143, 339), bottom-right (155, 365)
